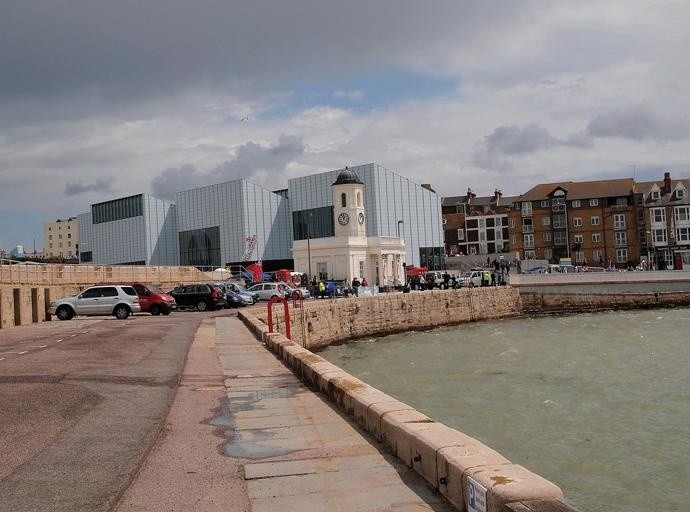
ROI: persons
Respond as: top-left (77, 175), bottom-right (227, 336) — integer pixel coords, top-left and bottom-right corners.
top-left (442, 272), bottom-right (451, 289)
top-left (309, 274), bottom-right (369, 299)
top-left (417, 274), bottom-right (427, 291)
top-left (448, 273), bottom-right (461, 289)
top-left (480, 252), bottom-right (510, 287)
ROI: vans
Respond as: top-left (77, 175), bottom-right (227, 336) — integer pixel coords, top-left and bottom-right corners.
top-left (246, 283), bottom-right (285, 303)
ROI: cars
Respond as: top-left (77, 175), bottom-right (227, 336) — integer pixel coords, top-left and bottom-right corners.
top-left (310, 281), bottom-right (348, 299)
top-left (280, 282), bottom-right (310, 300)
top-left (522, 267), bottom-right (548, 274)
top-left (460, 271), bottom-right (489, 289)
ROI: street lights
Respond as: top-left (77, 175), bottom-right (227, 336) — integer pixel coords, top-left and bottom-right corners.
top-left (456, 201), bottom-right (468, 255)
top-left (557, 203), bottom-right (569, 258)
top-left (398, 221), bottom-right (403, 239)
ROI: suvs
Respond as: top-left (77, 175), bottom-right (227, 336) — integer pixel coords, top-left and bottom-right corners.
top-left (425, 270), bottom-right (461, 290)
top-left (408, 277), bottom-right (422, 290)
top-left (47, 282), bottom-right (260, 320)
